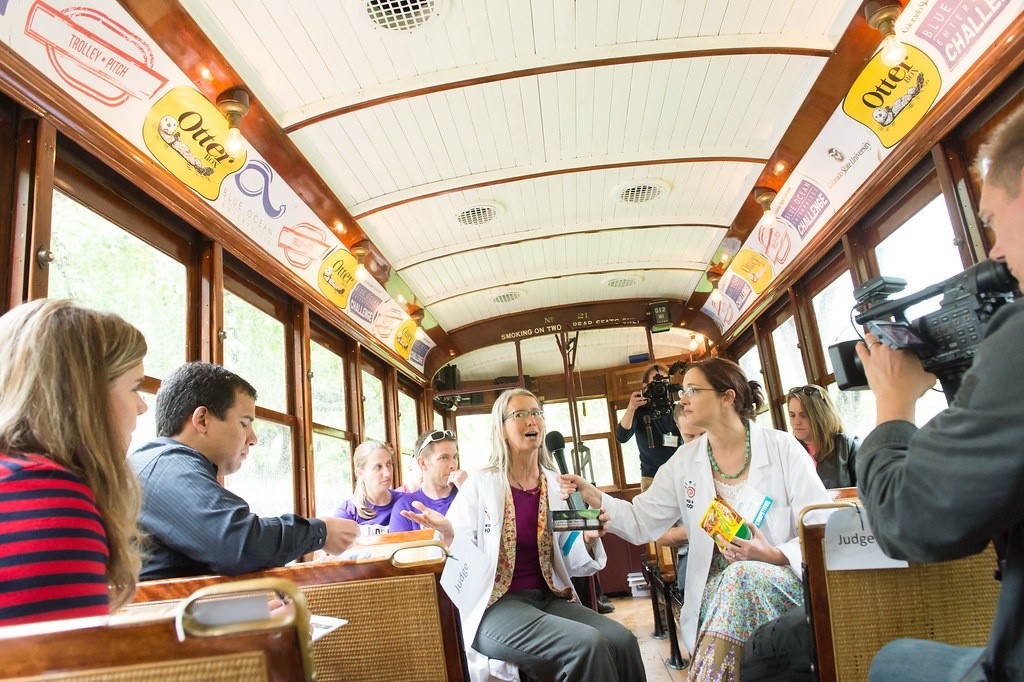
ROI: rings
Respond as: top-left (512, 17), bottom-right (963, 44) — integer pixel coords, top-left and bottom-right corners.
top-left (868, 341), bottom-right (879, 349)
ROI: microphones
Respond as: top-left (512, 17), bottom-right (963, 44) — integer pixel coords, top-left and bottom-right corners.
top-left (545, 431), bottom-right (587, 510)
top-left (669, 361), bottom-right (682, 375)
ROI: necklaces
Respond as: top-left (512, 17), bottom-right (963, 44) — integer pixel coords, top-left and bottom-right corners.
top-left (706, 423), bottom-right (750, 479)
top-left (509, 470), bottom-right (541, 495)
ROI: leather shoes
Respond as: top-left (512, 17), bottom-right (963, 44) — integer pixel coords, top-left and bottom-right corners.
top-left (598, 601), bottom-right (615, 613)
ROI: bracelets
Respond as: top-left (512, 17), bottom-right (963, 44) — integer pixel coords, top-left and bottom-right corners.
top-left (669, 528), bottom-right (676, 544)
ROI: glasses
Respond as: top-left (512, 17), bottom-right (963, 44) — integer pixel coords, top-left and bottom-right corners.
top-left (790, 387), bottom-right (827, 404)
top-left (678, 387), bottom-right (725, 398)
top-left (503, 411), bottom-right (543, 420)
top-left (417, 430), bottom-right (456, 453)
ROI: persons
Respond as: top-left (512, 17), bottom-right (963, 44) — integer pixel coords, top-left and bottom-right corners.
top-left (333, 441), bottom-right (410, 535)
top-left (854, 101), bottom-right (1024, 682)
top-left (616, 362), bottom-right (684, 494)
top-left (125, 361), bottom-right (360, 582)
top-left (388, 430), bottom-right (469, 533)
top-left (570, 574), bottom-right (615, 614)
top-left (655, 401), bottom-right (707, 603)
top-left (787, 383), bottom-right (860, 490)
top-left (558, 358), bottom-right (838, 682)
top-left (0, 298), bottom-right (147, 627)
top-left (400, 388), bottom-right (647, 682)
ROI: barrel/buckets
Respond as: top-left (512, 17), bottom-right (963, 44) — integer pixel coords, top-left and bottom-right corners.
top-left (627, 572), bottom-right (651, 597)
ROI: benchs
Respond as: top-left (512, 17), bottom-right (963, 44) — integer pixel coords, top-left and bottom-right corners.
top-left (639, 503), bottom-right (1001, 682)
top-left (0, 526), bottom-right (470, 682)
